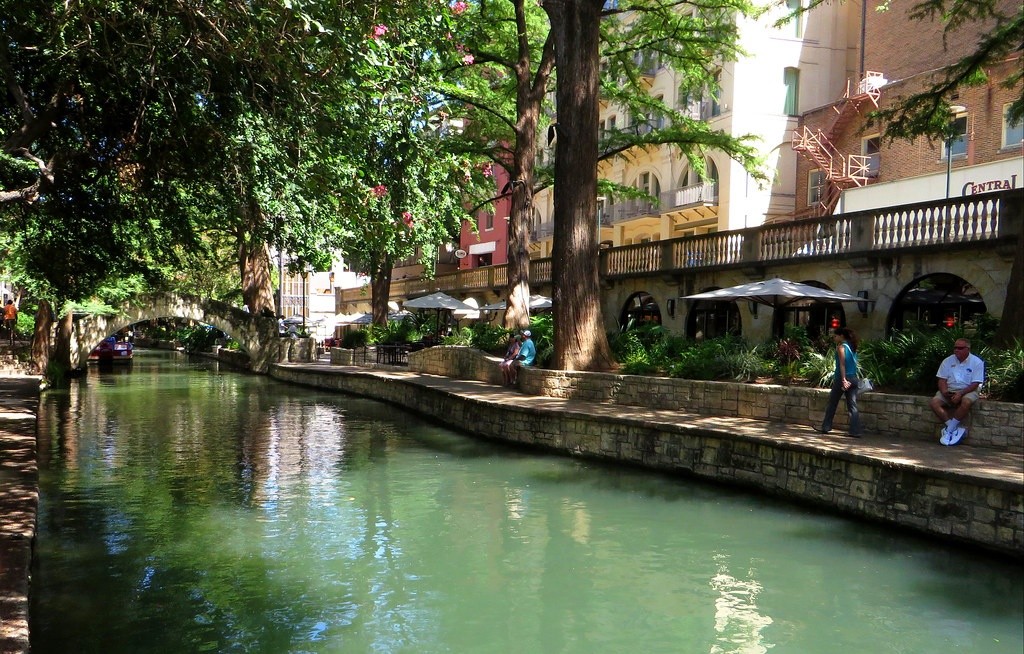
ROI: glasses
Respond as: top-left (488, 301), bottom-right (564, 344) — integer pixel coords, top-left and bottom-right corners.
top-left (953, 346), bottom-right (969, 350)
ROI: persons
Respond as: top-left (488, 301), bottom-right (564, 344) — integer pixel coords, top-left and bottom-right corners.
top-left (500, 330), bottom-right (536, 387)
top-left (2, 300), bottom-right (18, 340)
top-left (929, 338), bottom-right (985, 446)
top-left (812, 328), bottom-right (862, 438)
top-left (278, 314), bottom-right (297, 338)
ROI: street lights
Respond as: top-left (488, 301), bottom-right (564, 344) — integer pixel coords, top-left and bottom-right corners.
top-left (296, 269), bottom-right (314, 338)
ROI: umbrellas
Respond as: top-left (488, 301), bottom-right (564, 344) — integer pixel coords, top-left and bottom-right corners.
top-left (317, 310), bottom-right (415, 331)
top-left (281, 313), bottom-right (319, 331)
top-left (675, 278), bottom-right (878, 344)
top-left (402, 291), bottom-right (553, 346)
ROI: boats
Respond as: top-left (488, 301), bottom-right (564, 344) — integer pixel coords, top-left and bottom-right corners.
top-left (88, 336), bottom-right (134, 360)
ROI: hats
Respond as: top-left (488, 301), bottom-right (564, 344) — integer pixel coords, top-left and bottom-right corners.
top-left (509, 333), bottom-right (515, 339)
top-left (520, 329), bottom-right (531, 336)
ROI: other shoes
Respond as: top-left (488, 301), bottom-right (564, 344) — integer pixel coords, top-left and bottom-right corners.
top-left (510, 380), bottom-right (520, 384)
top-left (845, 432), bottom-right (862, 438)
top-left (503, 383), bottom-right (509, 389)
top-left (812, 423), bottom-right (828, 435)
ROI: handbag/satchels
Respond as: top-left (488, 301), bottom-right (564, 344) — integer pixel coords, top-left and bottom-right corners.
top-left (857, 378), bottom-right (873, 394)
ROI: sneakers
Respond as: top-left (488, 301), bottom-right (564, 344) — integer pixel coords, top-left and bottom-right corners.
top-left (940, 428), bottom-right (950, 445)
top-left (950, 427), bottom-right (965, 445)
